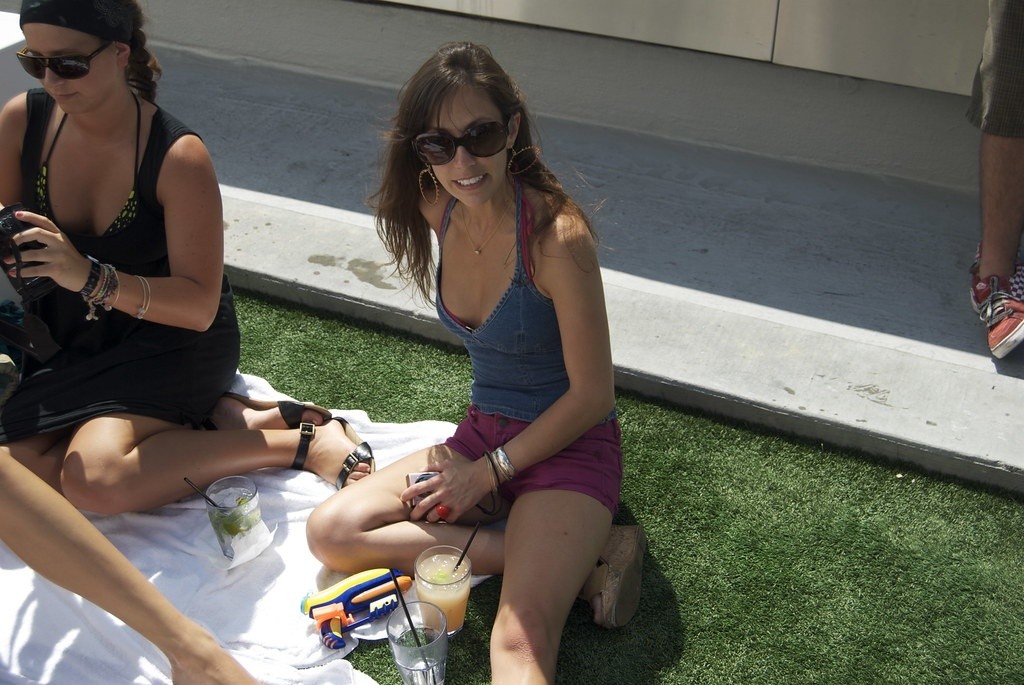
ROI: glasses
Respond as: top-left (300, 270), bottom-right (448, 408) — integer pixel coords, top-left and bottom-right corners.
top-left (17, 39), bottom-right (114, 79)
top-left (411, 113), bottom-right (511, 165)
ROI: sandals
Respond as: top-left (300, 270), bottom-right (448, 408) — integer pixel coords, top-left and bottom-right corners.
top-left (202, 392), bottom-right (332, 431)
top-left (291, 417), bottom-right (375, 490)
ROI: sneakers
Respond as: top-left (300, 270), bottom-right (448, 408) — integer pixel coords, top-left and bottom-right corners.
top-left (974, 265), bottom-right (1024, 359)
top-left (970, 240), bottom-right (1021, 315)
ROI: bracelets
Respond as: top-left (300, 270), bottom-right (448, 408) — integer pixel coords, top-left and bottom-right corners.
top-left (481, 449), bottom-right (517, 491)
top-left (78, 254), bottom-right (154, 321)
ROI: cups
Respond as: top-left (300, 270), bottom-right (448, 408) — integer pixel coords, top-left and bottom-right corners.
top-left (386, 601), bottom-right (447, 685)
top-left (206, 475), bottom-right (262, 560)
top-left (0, 203), bottom-right (60, 305)
top-left (414, 545), bottom-right (472, 638)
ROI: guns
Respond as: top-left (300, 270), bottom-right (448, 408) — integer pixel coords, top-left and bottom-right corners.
top-left (300, 567), bottom-right (413, 650)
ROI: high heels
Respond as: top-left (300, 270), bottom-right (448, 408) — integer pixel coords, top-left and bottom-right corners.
top-left (582, 525), bottom-right (647, 631)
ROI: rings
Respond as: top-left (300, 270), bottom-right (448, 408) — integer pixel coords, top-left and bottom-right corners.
top-left (437, 504), bottom-right (452, 518)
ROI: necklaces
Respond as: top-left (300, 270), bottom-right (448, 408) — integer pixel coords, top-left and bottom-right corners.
top-left (460, 198), bottom-right (512, 253)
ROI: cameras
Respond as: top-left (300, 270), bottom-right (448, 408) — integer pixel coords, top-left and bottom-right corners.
top-left (405, 472), bottom-right (441, 507)
top-left (0, 202), bottom-right (59, 303)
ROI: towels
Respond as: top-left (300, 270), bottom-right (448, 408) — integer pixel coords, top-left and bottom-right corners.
top-left (0, 368), bottom-right (492, 685)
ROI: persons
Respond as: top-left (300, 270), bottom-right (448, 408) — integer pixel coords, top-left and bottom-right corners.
top-left (0, 0), bottom-right (378, 516)
top-left (0, 447), bottom-right (269, 685)
top-left (965, 0), bottom-right (1024, 359)
top-left (306, 42), bottom-right (645, 685)
